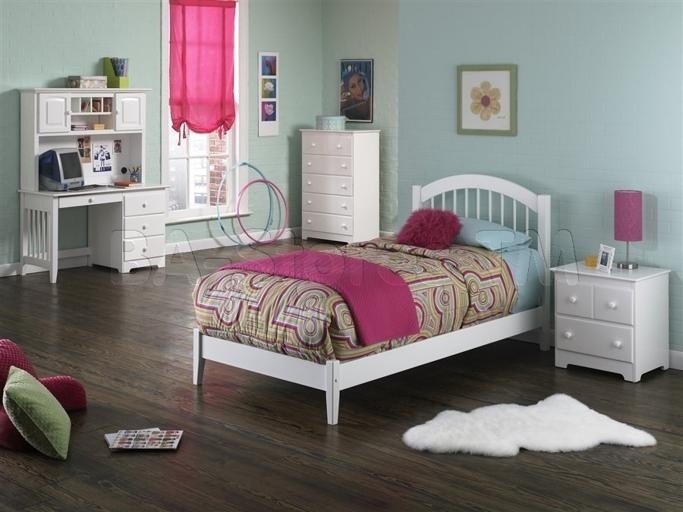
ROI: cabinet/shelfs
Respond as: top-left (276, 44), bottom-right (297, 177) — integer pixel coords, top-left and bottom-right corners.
top-left (298, 128), bottom-right (381, 244)
top-left (16, 87), bottom-right (152, 192)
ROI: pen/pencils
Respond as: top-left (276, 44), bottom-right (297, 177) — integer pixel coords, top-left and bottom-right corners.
top-left (129, 167), bottom-right (139, 174)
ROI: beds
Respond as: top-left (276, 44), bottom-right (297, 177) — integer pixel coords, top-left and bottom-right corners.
top-left (192, 173), bottom-right (551, 426)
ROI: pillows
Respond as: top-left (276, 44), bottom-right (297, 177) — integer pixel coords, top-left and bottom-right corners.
top-left (3, 370), bottom-right (71, 458)
top-left (453, 216), bottom-right (532, 252)
top-left (395, 204), bottom-right (463, 251)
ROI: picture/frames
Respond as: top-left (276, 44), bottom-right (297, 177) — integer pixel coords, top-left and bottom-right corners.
top-left (596, 242), bottom-right (617, 274)
top-left (334, 58), bottom-right (375, 126)
top-left (456, 64), bottom-right (518, 137)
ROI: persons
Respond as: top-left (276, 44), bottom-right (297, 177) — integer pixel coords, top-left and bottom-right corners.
top-left (340, 69), bottom-right (368, 120)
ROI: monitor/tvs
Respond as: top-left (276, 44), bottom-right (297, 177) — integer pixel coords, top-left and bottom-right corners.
top-left (39, 148), bottom-right (84, 190)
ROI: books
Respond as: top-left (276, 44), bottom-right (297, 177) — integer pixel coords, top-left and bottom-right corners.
top-left (101, 426), bottom-right (183, 453)
top-left (71, 98), bottom-right (115, 130)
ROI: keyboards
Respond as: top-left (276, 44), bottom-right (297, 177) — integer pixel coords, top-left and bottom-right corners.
top-left (70, 185), bottom-right (96, 192)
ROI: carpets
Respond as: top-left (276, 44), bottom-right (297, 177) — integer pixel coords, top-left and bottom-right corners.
top-left (400, 393), bottom-right (657, 458)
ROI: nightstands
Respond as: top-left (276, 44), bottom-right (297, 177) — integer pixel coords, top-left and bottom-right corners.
top-left (549, 259), bottom-right (672, 384)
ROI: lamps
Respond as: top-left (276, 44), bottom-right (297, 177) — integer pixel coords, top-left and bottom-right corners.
top-left (613, 190), bottom-right (642, 270)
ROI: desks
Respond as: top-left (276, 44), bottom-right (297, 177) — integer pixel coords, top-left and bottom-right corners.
top-left (17, 184), bottom-right (168, 284)
